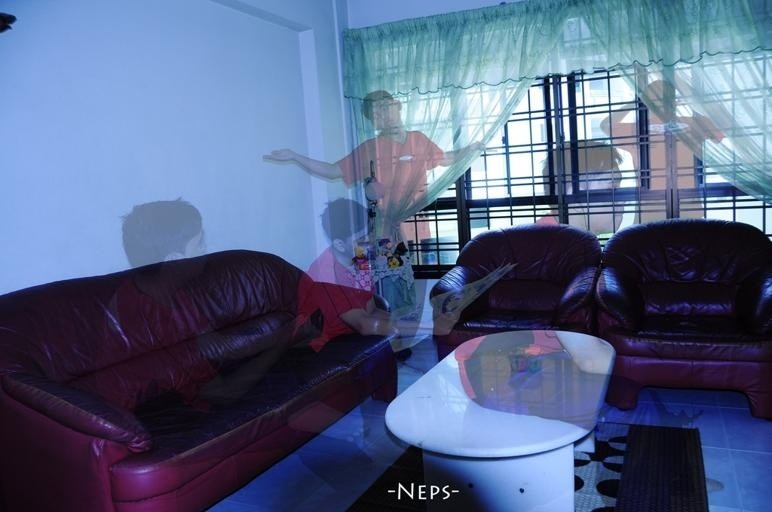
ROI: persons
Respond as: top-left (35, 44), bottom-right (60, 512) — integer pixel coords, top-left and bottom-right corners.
top-left (290, 198), bottom-right (461, 458)
top-left (92, 197), bottom-right (412, 512)
top-left (452, 139), bottom-right (726, 512)
top-left (599, 79), bottom-right (725, 226)
top-left (260, 90), bottom-right (498, 366)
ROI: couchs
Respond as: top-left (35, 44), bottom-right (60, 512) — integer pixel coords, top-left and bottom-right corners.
top-left (0, 250), bottom-right (398, 510)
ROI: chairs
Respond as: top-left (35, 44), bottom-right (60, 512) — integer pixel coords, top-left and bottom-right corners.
top-left (430, 219), bottom-right (771, 421)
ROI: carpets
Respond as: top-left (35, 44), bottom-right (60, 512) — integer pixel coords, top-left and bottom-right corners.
top-left (340, 421), bottom-right (708, 511)
